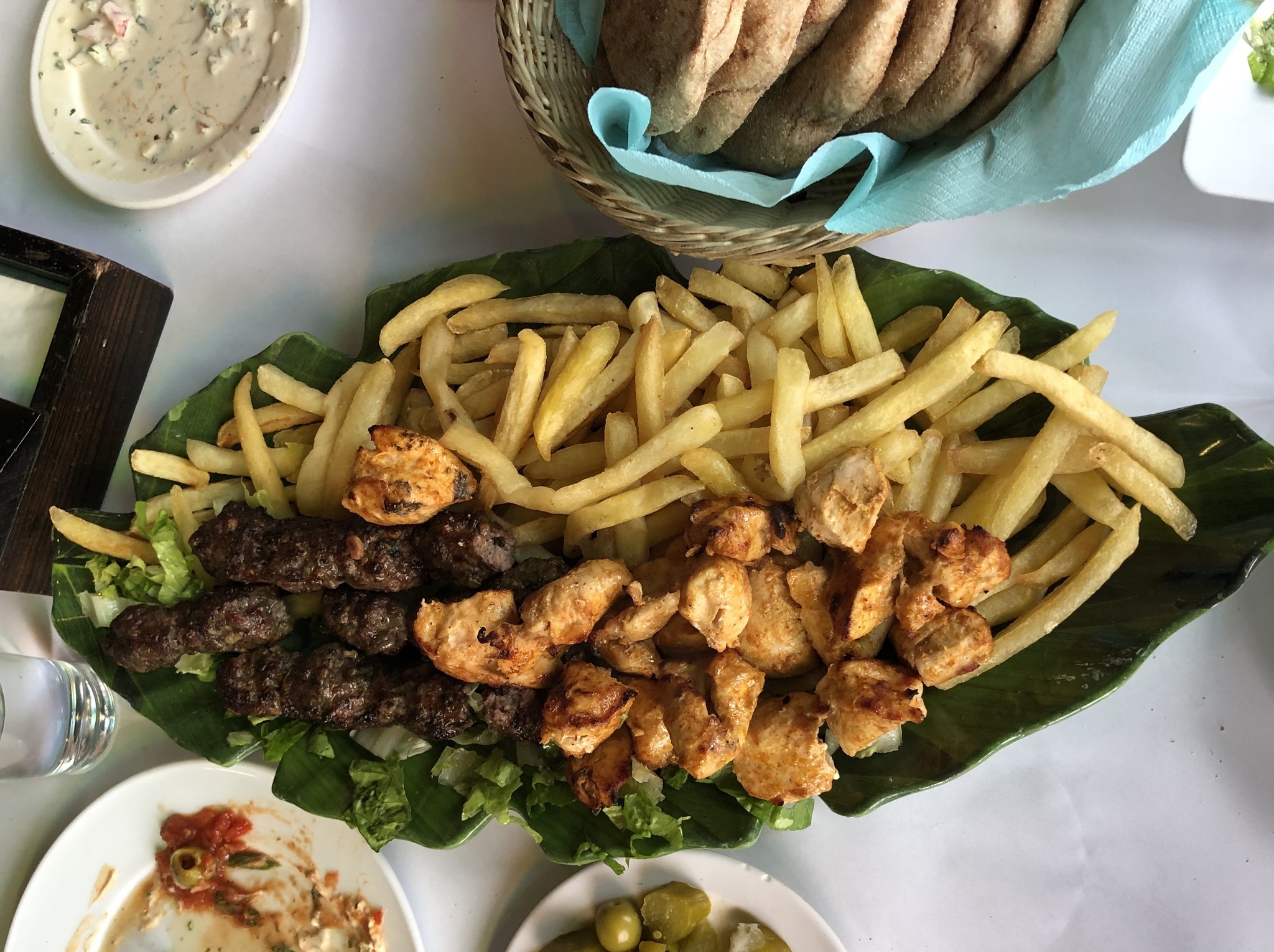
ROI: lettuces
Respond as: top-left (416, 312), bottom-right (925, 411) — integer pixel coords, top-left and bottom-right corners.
top-left (1241, 14), bottom-right (1274, 95)
top-left (84, 502), bottom-right (903, 877)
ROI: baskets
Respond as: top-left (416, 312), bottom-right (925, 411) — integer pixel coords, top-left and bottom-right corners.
top-left (496, 0), bottom-right (920, 259)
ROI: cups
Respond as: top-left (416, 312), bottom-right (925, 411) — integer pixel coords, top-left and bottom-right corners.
top-left (0, 650), bottom-right (117, 779)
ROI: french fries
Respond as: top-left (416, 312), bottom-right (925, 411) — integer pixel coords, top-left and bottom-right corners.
top-left (48, 255), bottom-right (1196, 693)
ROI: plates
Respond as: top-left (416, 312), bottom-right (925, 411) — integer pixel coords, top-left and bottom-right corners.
top-left (4, 761), bottom-right (426, 952)
top-left (504, 847), bottom-right (849, 952)
top-left (47, 236), bottom-right (1274, 866)
top-left (1181, 0), bottom-right (1271, 205)
top-left (29, 1), bottom-right (311, 209)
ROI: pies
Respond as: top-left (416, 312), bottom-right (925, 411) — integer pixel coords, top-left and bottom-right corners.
top-left (600, 0), bottom-right (1080, 177)
